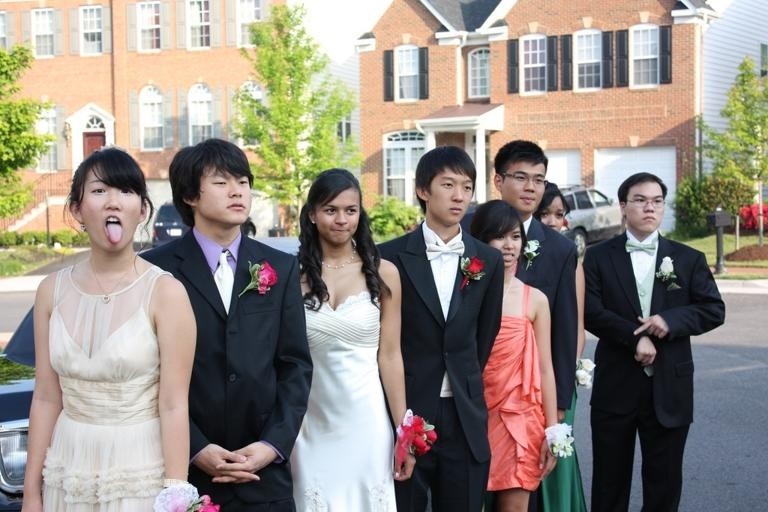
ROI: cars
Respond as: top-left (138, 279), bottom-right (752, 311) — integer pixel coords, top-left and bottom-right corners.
top-left (0, 236), bottom-right (303, 511)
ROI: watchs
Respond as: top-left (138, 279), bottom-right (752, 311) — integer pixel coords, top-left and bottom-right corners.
top-left (408, 444), bottom-right (416, 457)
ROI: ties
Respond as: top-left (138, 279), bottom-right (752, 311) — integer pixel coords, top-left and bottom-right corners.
top-left (213, 250), bottom-right (234, 315)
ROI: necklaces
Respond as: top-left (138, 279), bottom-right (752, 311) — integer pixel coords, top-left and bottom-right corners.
top-left (88, 260), bottom-right (131, 301)
top-left (321, 247), bottom-right (356, 268)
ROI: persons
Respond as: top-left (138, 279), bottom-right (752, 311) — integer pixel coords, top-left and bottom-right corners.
top-left (136, 138), bottom-right (312, 512)
top-left (582, 173), bottom-right (726, 512)
top-left (287, 167), bottom-right (416, 511)
top-left (373, 146), bottom-right (504, 511)
top-left (21, 144), bottom-right (199, 512)
top-left (532, 181), bottom-right (589, 512)
top-left (471, 200), bottom-right (559, 511)
top-left (460, 139), bottom-right (577, 512)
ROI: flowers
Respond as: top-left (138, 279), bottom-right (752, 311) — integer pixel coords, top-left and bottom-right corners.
top-left (153, 482), bottom-right (222, 512)
top-left (541, 420), bottom-right (576, 461)
top-left (237, 259), bottom-right (276, 298)
top-left (656, 257), bottom-right (679, 294)
top-left (576, 355), bottom-right (596, 390)
top-left (523, 239), bottom-right (542, 270)
top-left (395, 406), bottom-right (438, 473)
top-left (457, 256), bottom-right (483, 289)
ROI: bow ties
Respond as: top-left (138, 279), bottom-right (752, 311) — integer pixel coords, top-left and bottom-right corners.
top-left (625, 239), bottom-right (656, 256)
top-left (425, 241), bottom-right (465, 261)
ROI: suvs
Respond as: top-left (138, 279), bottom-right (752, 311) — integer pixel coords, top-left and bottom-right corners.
top-left (558, 182), bottom-right (627, 257)
top-left (152, 201), bottom-right (192, 249)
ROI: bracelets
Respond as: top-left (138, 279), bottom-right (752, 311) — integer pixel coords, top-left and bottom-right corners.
top-left (160, 479), bottom-right (186, 489)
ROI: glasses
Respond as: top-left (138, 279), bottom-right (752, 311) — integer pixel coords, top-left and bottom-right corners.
top-left (501, 173), bottom-right (547, 187)
top-left (626, 198), bottom-right (665, 208)
